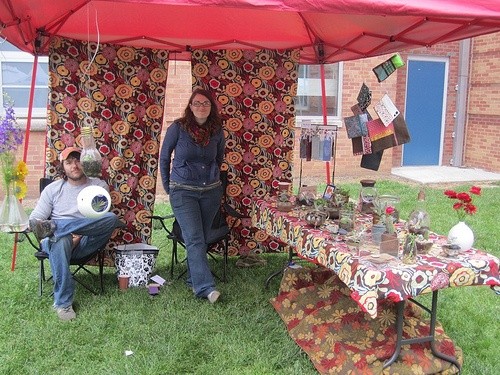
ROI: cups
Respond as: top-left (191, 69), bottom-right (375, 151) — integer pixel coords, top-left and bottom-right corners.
top-left (118, 275), bottom-right (131, 289)
top-left (353, 214), bottom-right (373, 255)
top-left (378, 195), bottom-right (401, 224)
top-left (338, 210), bottom-right (356, 232)
top-left (277, 181), bottom-right (290, 197)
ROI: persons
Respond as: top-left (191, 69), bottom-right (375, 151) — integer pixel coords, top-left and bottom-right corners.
top-left (27, 147), bottom-right (118, 321)
top-left (160, 89), bottom-right (226, 302)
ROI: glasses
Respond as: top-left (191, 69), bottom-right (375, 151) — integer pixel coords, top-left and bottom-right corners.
top-left (193, 100), bottom-right (210, 107)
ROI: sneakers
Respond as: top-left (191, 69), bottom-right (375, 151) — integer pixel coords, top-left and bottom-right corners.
top-left (29, 218), bottom-right (52, 242)
top-left (56, 305), bottom-right (76, 321)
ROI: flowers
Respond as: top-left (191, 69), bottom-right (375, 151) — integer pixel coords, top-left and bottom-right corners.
top-left (0, 90), bottom-right (28, 230)
top-left (444, 187), bottom-right (481, 221)
top-left (382, 208), bottom-right (396, 235)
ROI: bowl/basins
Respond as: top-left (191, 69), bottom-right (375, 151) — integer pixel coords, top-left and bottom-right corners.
top-left (279, 201), bottom-right (291, 212)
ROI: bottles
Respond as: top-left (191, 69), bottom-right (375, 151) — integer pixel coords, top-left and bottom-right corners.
top-left (80, 126), bottom-right (102, 179)
top-left (359, 179), bottom-right (378, 213)
top-left (408, 191), bottom-right (431, 242)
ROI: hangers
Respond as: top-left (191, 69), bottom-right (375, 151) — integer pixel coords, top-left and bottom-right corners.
top-left (303, 129), bottom-right (334, 140)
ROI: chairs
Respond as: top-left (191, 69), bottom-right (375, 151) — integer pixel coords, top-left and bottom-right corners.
top-left (34, 178), bottom-right (125, 297)
top-left (145, 170), bottom-right (248, 286)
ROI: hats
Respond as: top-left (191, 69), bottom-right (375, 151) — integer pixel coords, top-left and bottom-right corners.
top-left (60, 147), bottom-right (81, 164)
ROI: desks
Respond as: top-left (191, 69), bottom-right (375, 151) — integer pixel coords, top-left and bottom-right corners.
top-left (251, 195), bottom-right (500, 375)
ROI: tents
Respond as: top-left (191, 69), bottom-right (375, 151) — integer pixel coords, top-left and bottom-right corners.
top-left (0, 0), bottom-right (500, 271)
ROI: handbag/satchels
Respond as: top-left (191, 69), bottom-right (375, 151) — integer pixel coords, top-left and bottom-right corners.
top-left (113, 235), bottom-right (159, 287)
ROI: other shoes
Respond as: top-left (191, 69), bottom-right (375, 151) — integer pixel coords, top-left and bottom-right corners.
top-left (207, 291), bottom-right (221, 303)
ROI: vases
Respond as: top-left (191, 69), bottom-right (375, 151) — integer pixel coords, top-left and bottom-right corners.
top-left (448, 221), bottom-right (474, 251)
top-left (0, 194), bottom-right (29, 232)
top-left (380, 231), bottom-right (398, 257)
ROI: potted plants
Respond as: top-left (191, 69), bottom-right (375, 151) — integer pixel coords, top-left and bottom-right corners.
top-left (306, 187), bottom-right (356, 230)
top-left (370, 199), bottom-right (387, 242)
